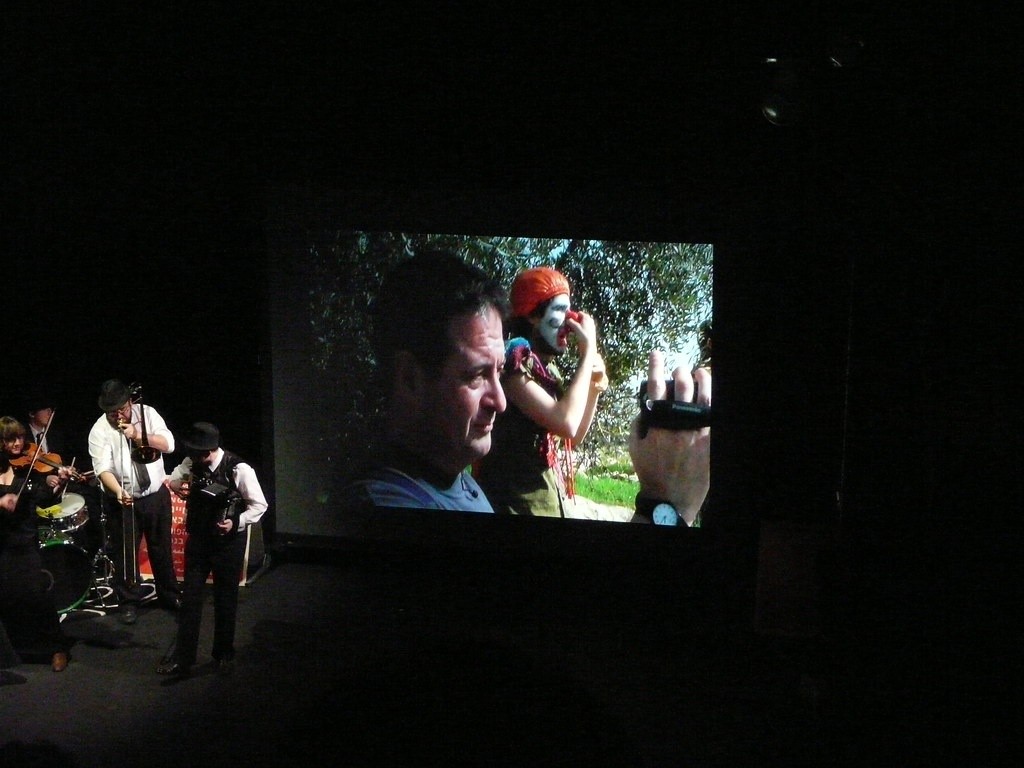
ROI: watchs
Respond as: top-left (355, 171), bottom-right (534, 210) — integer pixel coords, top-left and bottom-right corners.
top-left (635, 490), bottom-right (690, 527)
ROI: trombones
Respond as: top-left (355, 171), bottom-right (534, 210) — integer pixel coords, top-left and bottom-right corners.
top-left (117, 380), bottom-right (162, 585)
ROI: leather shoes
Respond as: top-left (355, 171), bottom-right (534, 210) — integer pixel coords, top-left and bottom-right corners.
top-left (211, 656), bottom-right (234, 675)
top-left (157, 661), bottom-right (189, 676)
top-left (52, 651), bottom-right (67, 671)
top-left (122, 605), bottom-right (138, 623)
top-left (158, 595), bottom-right (184, 611)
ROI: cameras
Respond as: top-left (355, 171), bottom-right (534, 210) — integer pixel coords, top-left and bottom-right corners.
top-left (637, 382), bottom-right (714, 440)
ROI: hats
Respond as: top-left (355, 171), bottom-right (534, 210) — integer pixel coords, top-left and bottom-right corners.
top-left (21, 394), bottom-right (53, 412)
top-left (97, 380), bottom-right (129, 411)
top-left (180, 423), bottom-right (218, 450)
top-left (510, 268), bottom-right (570, 317)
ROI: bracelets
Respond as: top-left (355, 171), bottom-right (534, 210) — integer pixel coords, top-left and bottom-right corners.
top-left (590, 374), bottom-right (609, 390)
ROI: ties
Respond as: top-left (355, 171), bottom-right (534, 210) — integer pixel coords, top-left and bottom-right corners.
top-left (124, 432), bottom-right (151, 493)
top-left (36, 433), bottom-right (40, 446)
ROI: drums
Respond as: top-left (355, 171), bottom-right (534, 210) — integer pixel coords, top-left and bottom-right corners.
top-left (35, 525), bottom-right (96, 615)
top-left (36, 491), bottom-right (90, 533)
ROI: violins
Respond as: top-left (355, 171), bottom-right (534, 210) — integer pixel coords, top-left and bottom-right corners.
top-left (8, 442), bottom-right (87, 484)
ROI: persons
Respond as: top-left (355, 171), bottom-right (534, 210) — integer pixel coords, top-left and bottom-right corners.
top-left (690, 321), bottom-right (711, 383)
top-left (248, 458), bottom-right (275, 509)
top-left (21, 396), bottom-right (153, 603)
top-left (341, 250), bottom-right (712, 529)
top-left (89, 379), bottom-right (175, 626)
top-left (0, 415), bottom-right (75, 672)
top-left (469, 267), bottom-right (609, 518)
top-left (155, 423), bottom-right (269, 672)
